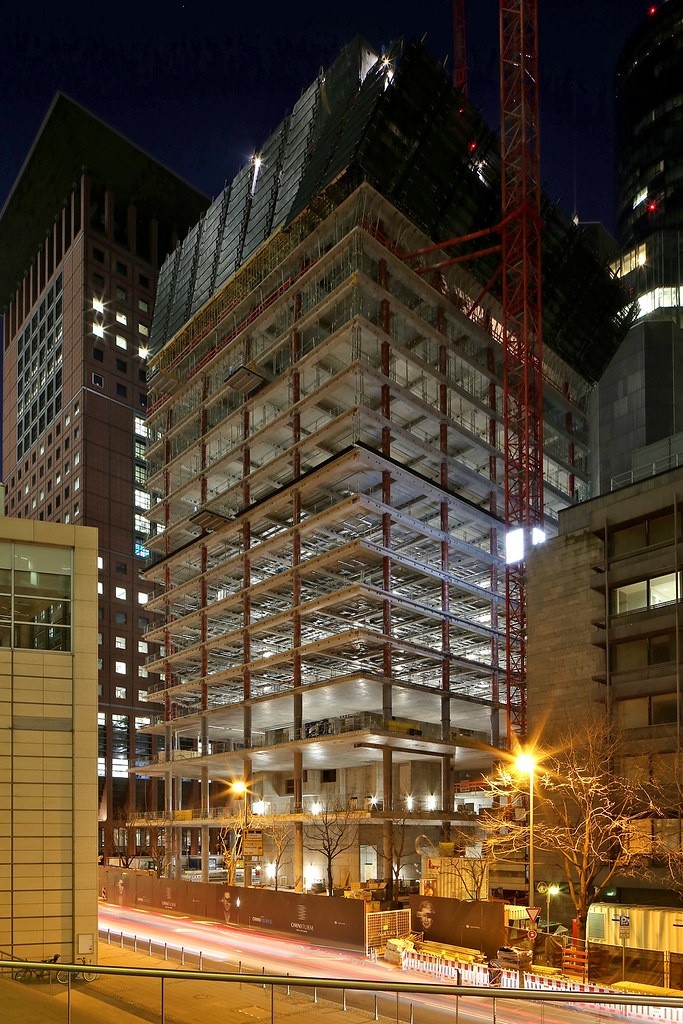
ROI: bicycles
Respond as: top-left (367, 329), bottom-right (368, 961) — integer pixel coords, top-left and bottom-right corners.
top-left (56, 957), bottom-right (98, 982)
top-left (16, 954), bottom-right (61, 981)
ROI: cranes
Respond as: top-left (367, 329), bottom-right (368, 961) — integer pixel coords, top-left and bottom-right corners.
top-left (452, 0), bottom-right (468, 98)
top-left (498, 0), bottom-right (545, 745)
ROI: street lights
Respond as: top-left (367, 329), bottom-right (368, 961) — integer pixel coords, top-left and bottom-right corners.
top-left (546, 883), bottom-right (559, 959)
top-left (234, 781), bottom-right (247, 890)
top-left (518, 747), bottom-right (539, 905)
